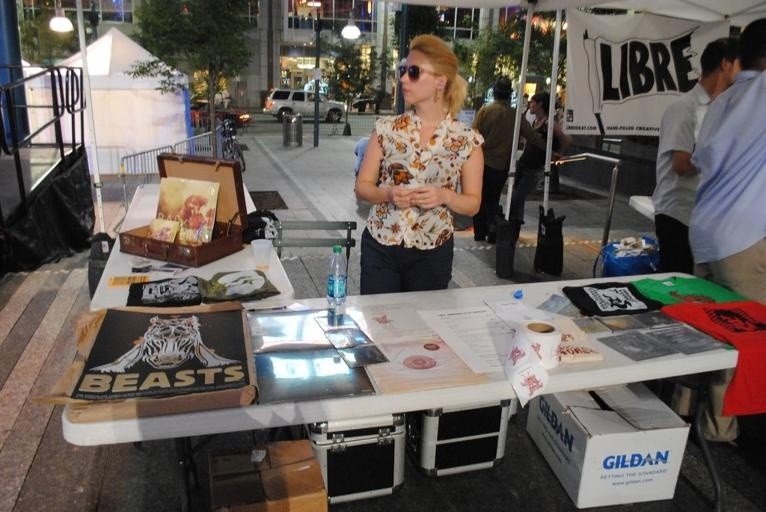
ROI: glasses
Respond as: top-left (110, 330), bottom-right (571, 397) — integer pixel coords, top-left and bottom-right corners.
top-left (399, 65), bottom-right (441, 80)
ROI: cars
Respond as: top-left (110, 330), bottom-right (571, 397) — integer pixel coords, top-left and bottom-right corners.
top-left (484, 87), bottom-right (519, 113)
top-left (191, 99), bottom-right (252, 126)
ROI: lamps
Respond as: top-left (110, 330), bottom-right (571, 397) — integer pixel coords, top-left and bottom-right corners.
top-left (342, 1), bottom-right (361, 40)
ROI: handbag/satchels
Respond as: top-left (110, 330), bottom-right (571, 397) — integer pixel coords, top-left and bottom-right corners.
top-left (535, 204), bottom-right (565, 276)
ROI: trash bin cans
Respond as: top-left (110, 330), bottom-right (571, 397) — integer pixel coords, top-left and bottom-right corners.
top-left (282, 112), bottom-right (302, 146)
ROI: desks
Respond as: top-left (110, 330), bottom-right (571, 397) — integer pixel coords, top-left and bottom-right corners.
top-left (629, 196), bottom-right (655, 222)
top-left (88, 183), bottom-right (294, 312)
top-left (62, 272), bottom-right (739, 512)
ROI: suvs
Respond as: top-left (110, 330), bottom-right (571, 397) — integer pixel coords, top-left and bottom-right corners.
top-left (263, 88), bottom-right (346, 124)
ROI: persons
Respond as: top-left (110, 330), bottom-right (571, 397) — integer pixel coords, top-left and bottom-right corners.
top-left (355, 35), bottom-right (484, 295)
top-left (472, 76), bottom-right (571, 244)
top-left (650, 38), bottom-right (746, 274)
top-left (689, 16), bottom-right (766, 443)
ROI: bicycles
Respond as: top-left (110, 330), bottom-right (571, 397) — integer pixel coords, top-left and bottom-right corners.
top-left (218, 116), bottom-right (247, 172)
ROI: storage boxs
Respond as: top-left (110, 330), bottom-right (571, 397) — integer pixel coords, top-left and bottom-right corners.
top-left (527, 381), bottom-right (691, 508)
top-left (406, 401), bottom-right (511, 480)
top-left (209, 438), bottom-right (329, 511)
top-left (304, 413), bottom-right (406, 504)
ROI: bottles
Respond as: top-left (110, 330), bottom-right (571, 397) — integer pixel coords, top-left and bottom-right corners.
top-left (325, 245), bottom-right (348, 305)
top-left (325, 306), bottom-right (346, 365)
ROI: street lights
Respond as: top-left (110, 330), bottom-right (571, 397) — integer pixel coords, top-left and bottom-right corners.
top-left (311, 7), bottom-right (361, 147)
